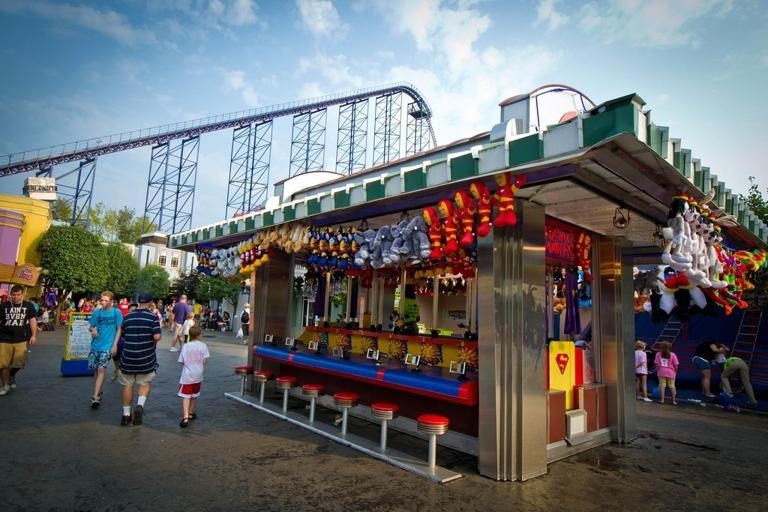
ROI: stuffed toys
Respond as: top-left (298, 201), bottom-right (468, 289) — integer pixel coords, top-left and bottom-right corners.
top-left (194, 171), bottom-right (529, 297)
top-left (632, 190), bottom-right (768, 318)
top-left (546, 266), bottom-right (594, 313)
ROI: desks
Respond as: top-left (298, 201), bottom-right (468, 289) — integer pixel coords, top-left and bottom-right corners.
top-left (253, 341), bottom-right (479, 407)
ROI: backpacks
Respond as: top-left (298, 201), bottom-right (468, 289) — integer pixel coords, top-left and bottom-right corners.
top-left (241, 309), bottom-right (250, 323)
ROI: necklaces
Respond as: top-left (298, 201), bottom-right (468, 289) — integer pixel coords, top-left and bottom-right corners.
top-left (11, 296), bottom-right (23, 317)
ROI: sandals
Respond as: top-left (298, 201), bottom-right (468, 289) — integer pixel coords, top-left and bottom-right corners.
top-left (188, 413), bottom-right (197, 421)
top-left (179, 417), bottom-right (189, 428)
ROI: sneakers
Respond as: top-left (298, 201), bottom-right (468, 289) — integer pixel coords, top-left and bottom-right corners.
top-left (168, 346), bottom-right (179, 353)
top-left (719, 392), bottom-right (735, 400)
top-left (121, 411), bottom-right (133, 426)
top-left (704, 393), bottom-right (718, 398)
top-left (111, 368), bottom-right (121, 382)
top-left (0, 383), bottom-right (11, 396)
top-left (643, 397), bottom-right (654, 402)
top-left (636, 394), bottom-right (644, 400)
top-left (9, 376), bottom-right (18, 390)
top-left (89, 391), bottom-right (104, 409)
top-left (132, 405), bottom-right (144, 426)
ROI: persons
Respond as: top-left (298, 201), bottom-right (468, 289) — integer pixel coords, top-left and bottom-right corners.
top-left (29, 290), bottom-right (231, 428)
top-left (720, 355), bottom-right (758, 406)
top-left (0, 285), bottom-right (38, 397)
top-left (652, 340), bottom-right (680, 406)
top-left (690, 338), bottom-right (728, 398)
top-left (574, 338), bottom-right (592, 351)
top-left (634, 340), bottom-right (652, 403)
top-left (238, 302), bottom-right (249, 345)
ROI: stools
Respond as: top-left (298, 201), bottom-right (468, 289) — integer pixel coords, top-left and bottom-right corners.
top-left (417, 413), bottom-right (449, 469)
top-left (254, 370), bottom-right (272, 402)
top-left (235, 366), bottom-right (255, 396)
top-left (302, 384), bottom-right (325, 422)
top-left (276, 376), bottom-right (298, 412)
top-left (371, 403), bottom-right (400, 450)
top-left (334, 392), bottom-right (359, 435)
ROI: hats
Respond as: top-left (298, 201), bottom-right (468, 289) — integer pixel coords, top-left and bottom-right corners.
top-left (127, 302), bottom-right (139, 309)
top-left (137, 293), bottom-right (155, 304)
